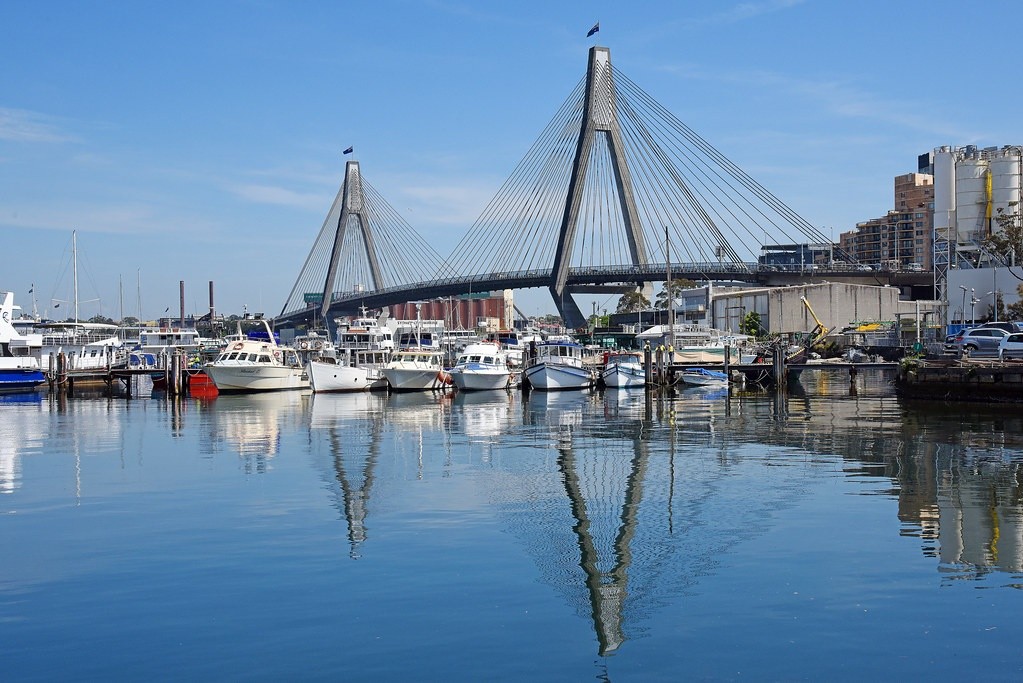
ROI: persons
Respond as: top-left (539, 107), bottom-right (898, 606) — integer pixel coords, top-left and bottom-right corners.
top-left (668, 342), bottom-right (675, 365)
top-left (194, 355), bottom-right (200, 363)
top-left (655, 341), bottom-right (665, 352)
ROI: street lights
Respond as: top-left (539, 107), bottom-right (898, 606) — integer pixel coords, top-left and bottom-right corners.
top-left (830, 227), bottom-right (833, 262)
top-left (959, 285), bottom-right (967, 328)
top-left (812, 226), bottom-right (825, 276)
top-left (699, 237), bottom-right (701, 272)
top-left (970, 288), bottom-right (980, 328)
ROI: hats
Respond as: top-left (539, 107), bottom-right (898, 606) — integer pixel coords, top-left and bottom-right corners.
top-left (598, 373), bottom-right (603, 377)
top-left (658, 340), bottom-right (661, 343)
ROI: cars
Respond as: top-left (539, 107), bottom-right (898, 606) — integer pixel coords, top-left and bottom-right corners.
top-left (944, 321), bottom-right (1023, 362)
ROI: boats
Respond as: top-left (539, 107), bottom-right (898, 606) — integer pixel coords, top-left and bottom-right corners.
top-left (603, 386), bottom-right (645, 421)
top-left (1, 230), bottom-right (512, 393)
top-left (680, 369), bottom-right (729, 385)
top-left (527, 386), bottom-right (597, 435)
top-left (602, 348), bottom-right (646, 388)
top-left (522, 336), bottom-right (600, 390)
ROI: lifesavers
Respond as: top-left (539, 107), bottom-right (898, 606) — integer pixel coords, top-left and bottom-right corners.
top-left (315, 341), bottom-right (322, 349)
top-left (301, 341), bottom-right (308, 349)
top-left (618, 349), bottom-right (627, 354)
top-left (234, 342), bottom-right (244, 350)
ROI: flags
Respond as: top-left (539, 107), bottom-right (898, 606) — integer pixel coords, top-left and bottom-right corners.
top-left (29, 289), bottom-right (33, 293)
top-left (55, 304), bottom-right (59, 308)
